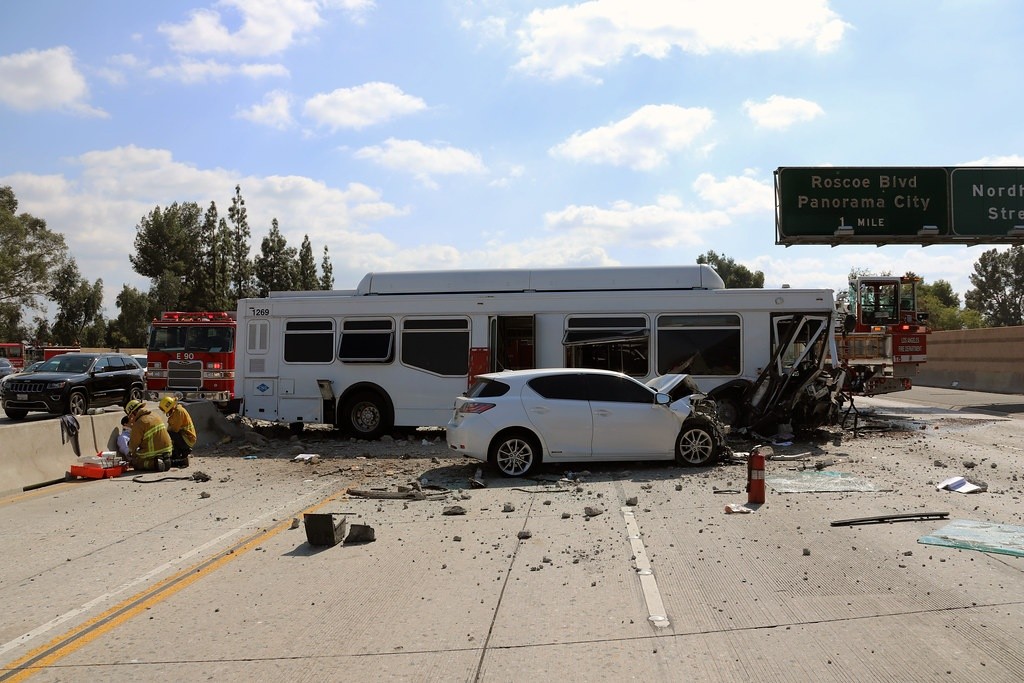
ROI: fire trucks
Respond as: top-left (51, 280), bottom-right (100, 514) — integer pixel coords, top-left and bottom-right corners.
top-left (143, 310), bottom-right (240, 407)
top-left (772, 275), bottom-right (931, 392)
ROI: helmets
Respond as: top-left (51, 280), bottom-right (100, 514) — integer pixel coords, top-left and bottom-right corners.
top-left (159, 396), bottom-right (178, 414)
top-left (125, 399), bottom-right (146, 418)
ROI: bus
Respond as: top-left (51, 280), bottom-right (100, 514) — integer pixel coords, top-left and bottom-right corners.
top-left (232, 265), bottom-right (885, 444)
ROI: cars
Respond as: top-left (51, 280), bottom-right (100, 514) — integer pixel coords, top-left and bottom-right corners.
top-left (0, 354), bottom-right (148, 379)
top-left (445, 369), bottom-right (727, 479)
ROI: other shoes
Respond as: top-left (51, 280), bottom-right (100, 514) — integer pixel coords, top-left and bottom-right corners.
top-left (162, 452), bottom-right (171, 471)
top-left (154, 455), bottom-right (165, 471)
top-left (181, 447), bottom-right (192, 458)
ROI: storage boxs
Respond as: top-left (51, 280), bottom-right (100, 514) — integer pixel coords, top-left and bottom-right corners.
top-left (71, 466), bottom-right (121, 478)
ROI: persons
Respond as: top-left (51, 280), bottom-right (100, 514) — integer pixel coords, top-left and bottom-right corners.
top-left (117, 396), bottom-right (197, 472)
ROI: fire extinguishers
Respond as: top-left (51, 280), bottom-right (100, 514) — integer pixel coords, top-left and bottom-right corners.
top-left (747, 444), bottom-right (766, 504)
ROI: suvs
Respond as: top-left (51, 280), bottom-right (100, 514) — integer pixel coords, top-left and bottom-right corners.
top-left (0, 353), bottom-right (146, 421)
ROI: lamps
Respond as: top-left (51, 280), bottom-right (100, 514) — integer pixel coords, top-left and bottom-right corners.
top-left (1007, 225), bottom-right (1024, 237)
top-left (833, 224), bottom-right (854, 240)
top-left (917, 226), bottom-right (939, 237)
